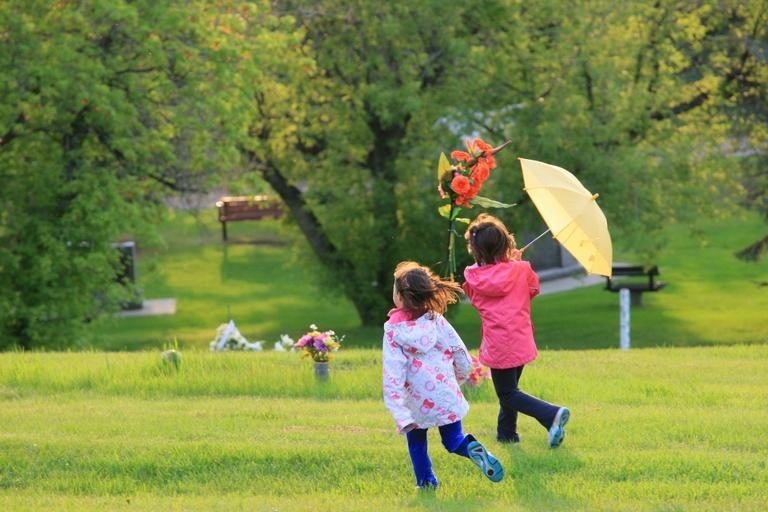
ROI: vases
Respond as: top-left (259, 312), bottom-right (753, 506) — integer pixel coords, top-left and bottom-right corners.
top-left (314, 359), bottom-right (329, 382)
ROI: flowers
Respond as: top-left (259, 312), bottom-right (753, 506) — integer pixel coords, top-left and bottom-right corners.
top-left (437, 137), bottom-right (518, 283)
top-left (294, 324), bottom-right (346, 363)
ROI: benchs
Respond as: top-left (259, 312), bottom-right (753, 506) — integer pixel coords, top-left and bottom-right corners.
top-left (217, 196), bottom-right (284, 241)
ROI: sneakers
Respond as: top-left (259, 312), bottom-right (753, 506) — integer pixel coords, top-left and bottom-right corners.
top-left (466, 439), bottom-right (505, 484)
top-left (547, 408), bottom-right (570, 448)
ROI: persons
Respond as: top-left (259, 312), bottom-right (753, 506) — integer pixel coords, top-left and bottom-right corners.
top-left (461, 213), bottom-right (571, 450)
top-left (378, 259), bottom-right (504, 492)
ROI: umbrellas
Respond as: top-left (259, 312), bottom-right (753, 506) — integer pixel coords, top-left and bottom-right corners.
top-left (516, 156), bottom-right (615, 282)
